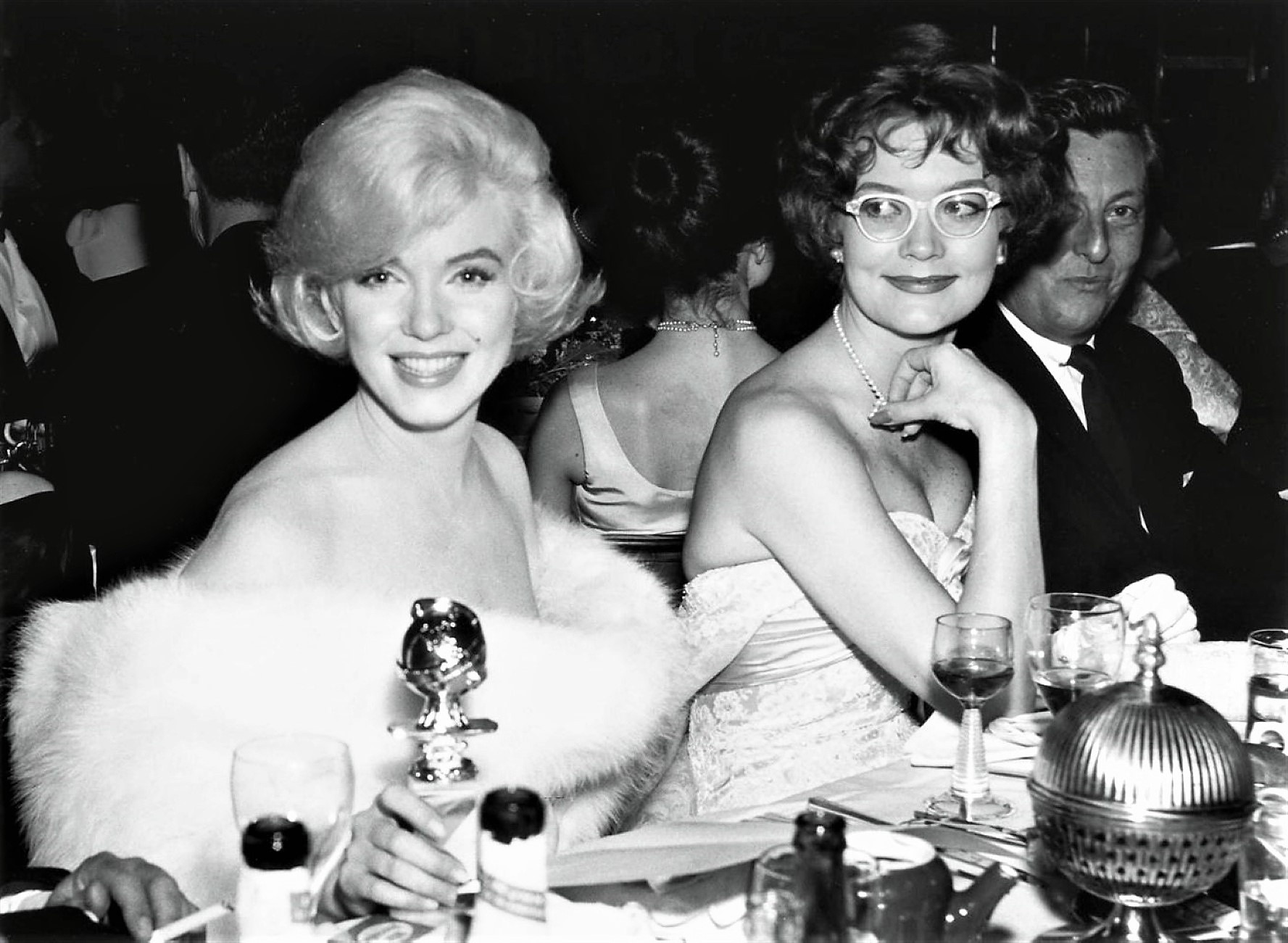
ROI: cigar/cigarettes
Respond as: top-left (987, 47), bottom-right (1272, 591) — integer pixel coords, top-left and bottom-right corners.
top-left (140, 898), bottom-right (235, 942)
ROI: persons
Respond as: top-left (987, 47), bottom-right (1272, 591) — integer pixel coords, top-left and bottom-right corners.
top-left (5, 69), bottom-right (688, 920)
top-left (952, 74), bottom-right (1287, 641)
top-left (531, 114), bottom-right (797, 545)
top-left (670, 19), bottom-right (1053, 822)
top-left (1, 39), bottom-right (357, 590)
top-left (0, 850), bottom-right (205, 943)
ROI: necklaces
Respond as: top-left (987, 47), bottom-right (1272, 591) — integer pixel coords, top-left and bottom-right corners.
top-left (656, 320), bottom-right (758, 354)
top-left (831, 302), bottom-right (957, 429)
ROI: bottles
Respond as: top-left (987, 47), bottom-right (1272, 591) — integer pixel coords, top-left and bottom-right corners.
top-left (785, 809), bottom-right (852, 943)
top-left (233, 810), bottom-right (318, 943)
top-left (463, 788), bottom-right (555, 943)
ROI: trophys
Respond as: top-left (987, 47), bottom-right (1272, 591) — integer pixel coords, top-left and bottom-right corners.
top-left (384, 592), bottom-right (501, 900)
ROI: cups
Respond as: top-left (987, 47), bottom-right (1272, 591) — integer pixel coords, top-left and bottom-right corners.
top-left (1245, 628), bottom-right (1288, 722)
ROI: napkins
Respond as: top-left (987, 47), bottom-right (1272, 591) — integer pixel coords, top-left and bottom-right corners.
top-left (907, 710), bottom-right (1043, 768)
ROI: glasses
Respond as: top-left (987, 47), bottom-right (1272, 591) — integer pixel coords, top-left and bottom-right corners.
top-left (827, 189), bottom-right (1016, 244)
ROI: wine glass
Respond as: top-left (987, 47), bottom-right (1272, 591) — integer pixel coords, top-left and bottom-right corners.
top-left (1028, 592), bottom-right (1125, 717)
top-left (232, 731), bottom-right (354, 880)
top-left (739, 846), bottom-right (889, 943)
top-left (929, 609), bottom-right (1014, 709)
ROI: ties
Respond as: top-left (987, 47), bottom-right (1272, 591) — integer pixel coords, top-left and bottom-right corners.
top-left (1064, 342), bottom-right (1145, 540)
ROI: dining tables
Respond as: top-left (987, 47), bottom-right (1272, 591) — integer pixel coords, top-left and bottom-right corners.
top-left (547, 640), bottom-right (1287, 943)
